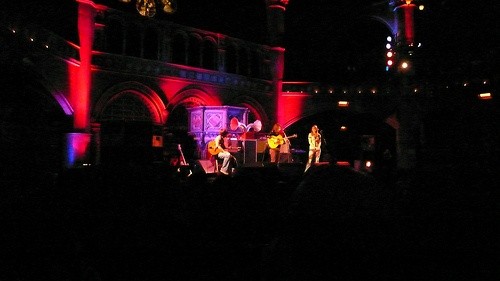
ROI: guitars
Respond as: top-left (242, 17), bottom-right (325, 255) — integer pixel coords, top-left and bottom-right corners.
top-left (267, 134), bottom-right (297, 149)
top-left (208, 140), bottom-right (245, 156)
top-left (178, 144), bottom-right (192, 176)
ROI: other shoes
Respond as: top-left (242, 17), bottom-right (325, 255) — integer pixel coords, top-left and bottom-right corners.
top-left (220, 168), bottom-right (229, 175)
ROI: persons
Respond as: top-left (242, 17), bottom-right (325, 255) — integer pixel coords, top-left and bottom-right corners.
top-left (267, 123), bottom-right (285, 164)
top-left (308, 125), bottom-right (321, 164)
top-left (215, 130), bottom-right (237, 175)
